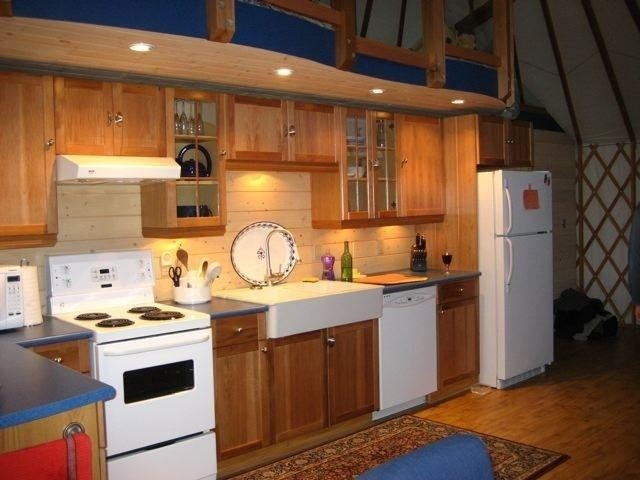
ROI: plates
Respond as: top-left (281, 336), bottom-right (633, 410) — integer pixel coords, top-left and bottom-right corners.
top-left (229, 221), bottom-right (296, 287)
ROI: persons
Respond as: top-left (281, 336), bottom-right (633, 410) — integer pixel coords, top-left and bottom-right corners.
top-left (628, 204), bottom-right (640, 321)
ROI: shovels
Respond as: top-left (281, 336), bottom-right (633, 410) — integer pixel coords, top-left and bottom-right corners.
top-left (176, 249), bottom-right (190, 272)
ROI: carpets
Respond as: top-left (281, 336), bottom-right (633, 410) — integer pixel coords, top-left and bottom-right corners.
top-left (216, 413), bottom-right (571, 480)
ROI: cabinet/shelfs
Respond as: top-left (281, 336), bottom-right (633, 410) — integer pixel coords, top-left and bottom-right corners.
top-left (25, 338), bottom-right (92, 379)
top-left (443, 115), bottom-right (534, 173)
top-left (53, 72), bottom-right (167, 158)
top-left (1, 64), bottom-right (59, 250)
top-left (311, 107), bottom-right (397, 229)
top-left (273, 318), bottom-right (380, 445)
top-left (138, 87), bottom-right (226, 240)
top-left (0, 397), bottom-right (109, 480)
top-left (397, 113), bottom-right (446, 223)
top-left (212, 310), bottom-right (272, 461)
top-left (225, 93), bottom-right (340, 171)
top-left (427, 275), bottom-right (480, 405)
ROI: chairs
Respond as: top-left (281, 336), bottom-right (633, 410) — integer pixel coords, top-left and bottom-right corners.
top-left (352, 434), bottom-right (495, 480)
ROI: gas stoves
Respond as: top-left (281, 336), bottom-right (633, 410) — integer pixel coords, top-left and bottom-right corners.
top-left (53, 302), bottom-right (211, 345)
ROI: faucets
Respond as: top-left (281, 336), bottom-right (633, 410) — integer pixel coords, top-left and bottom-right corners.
top-left (264, 229), bottom-right (288, 286)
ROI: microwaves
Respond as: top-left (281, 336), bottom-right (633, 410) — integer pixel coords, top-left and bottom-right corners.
top-left (0, 271), bottom-right (25, 332)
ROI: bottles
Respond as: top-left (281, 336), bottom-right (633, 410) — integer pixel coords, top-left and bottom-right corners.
top-left (340, 240), bottom-right (354, 283)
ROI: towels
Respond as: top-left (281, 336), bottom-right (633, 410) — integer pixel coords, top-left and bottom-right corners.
top-left (0, 433), bottom-right (93, 480)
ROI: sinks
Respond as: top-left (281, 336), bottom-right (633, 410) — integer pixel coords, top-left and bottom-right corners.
top-left (210, 279), bottom-right (385, 339)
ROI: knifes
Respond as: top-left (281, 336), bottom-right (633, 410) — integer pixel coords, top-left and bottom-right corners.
top-left (415, 233), bottom-right (426, 250)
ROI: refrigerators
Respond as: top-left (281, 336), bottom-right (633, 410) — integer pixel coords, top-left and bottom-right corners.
top-left (476, 168), bottom-right (554, 391)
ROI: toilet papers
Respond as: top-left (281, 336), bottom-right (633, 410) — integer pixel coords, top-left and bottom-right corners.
top-left (20, 265), bottom-right (43, 326)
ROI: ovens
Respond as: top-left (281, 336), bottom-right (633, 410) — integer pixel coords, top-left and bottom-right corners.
top-left (96, 328), bottom-right (217, 458)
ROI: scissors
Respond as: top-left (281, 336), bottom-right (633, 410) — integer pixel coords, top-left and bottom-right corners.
top-left (169, 267), bottom-right (182, 287)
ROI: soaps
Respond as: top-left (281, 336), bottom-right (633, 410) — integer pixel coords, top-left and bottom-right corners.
top-left (302, 277), bottom-right (319, 283)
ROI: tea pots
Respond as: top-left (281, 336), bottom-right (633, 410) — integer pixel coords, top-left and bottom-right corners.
top-left (177, 144), bottom-right (212, 178)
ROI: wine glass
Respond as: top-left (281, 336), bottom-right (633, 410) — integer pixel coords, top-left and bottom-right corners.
top-left (174, 99), bottom-right (206, 136)
top-left (438, 249), bottom-right (455, 276)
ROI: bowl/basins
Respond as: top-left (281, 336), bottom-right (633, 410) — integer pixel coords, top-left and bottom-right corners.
top-left (347, 165), bottom-right (366, 178)
